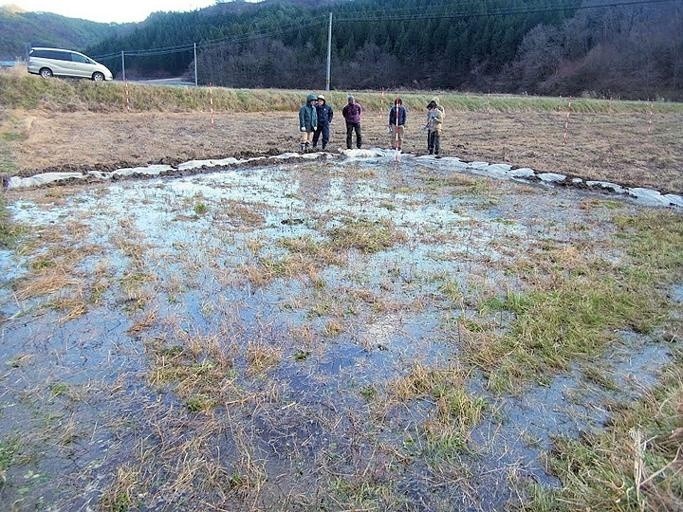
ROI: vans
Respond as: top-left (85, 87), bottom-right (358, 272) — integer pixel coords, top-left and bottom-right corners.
top-left (27, 47), bottom-right (114, 82)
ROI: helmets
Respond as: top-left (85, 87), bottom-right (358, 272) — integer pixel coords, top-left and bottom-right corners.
top-left (307, 94), bottom-right (326, 106)
top-left (348, 97), bottom-right (354, 100)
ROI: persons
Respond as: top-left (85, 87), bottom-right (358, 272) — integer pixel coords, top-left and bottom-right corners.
top-left (388, 97), bottom-right (407, 151)
top-left (425, 96), bottom-right (445, 155)
top-left (342, 96), bottom-right (362, 149)
top-left (299, 94), bottom-right (333, 150)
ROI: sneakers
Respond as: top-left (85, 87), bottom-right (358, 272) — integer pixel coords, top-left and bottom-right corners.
top-left (357, 145), bottom-right (362, 149)
top-left (347, 145), bottom-right (352, 148)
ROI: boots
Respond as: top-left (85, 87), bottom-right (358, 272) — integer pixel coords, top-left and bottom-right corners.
top-left (429, 149), bottom-right (439, 155)
top-left (300, 142), bottom-right (327, 154)
